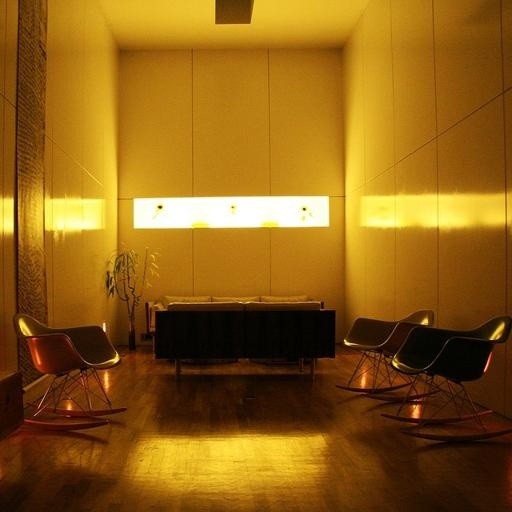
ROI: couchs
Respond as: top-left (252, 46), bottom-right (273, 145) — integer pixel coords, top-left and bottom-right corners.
top-left (145, 295), bottom-right (325, 357)
top-left (155, 301), bottom-right (336, 381)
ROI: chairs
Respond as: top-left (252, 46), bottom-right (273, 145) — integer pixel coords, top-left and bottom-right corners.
top-left (336, 310), bottom-right (442, 401)
top-left (381, 316), bottom-right (511, 442)
top-left (14, 314), bottom-right (127, 431)
